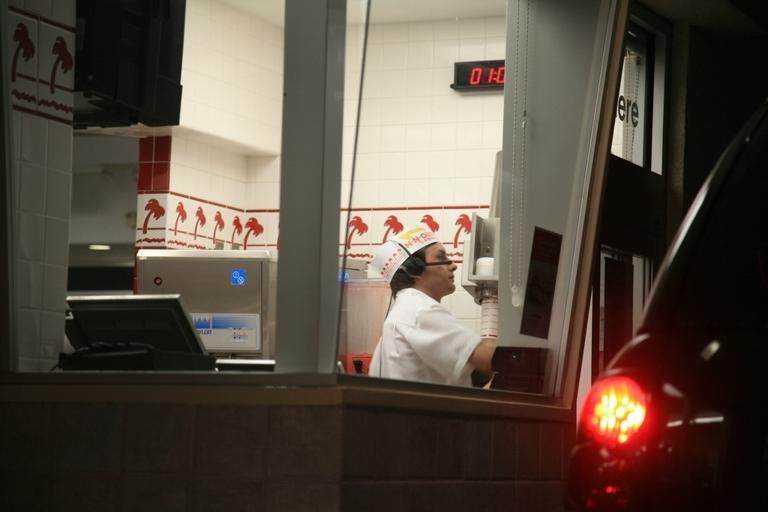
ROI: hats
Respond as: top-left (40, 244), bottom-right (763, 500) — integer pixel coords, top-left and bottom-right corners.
top-left (370, 222), bottom-right (440, 283)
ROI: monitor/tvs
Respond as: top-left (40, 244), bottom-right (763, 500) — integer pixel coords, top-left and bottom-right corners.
top-left (66, 294), bottom-right (209, 357)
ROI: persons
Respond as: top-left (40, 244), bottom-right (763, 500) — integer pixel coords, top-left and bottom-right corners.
top-left (367, 225), bottom-right (507, 387)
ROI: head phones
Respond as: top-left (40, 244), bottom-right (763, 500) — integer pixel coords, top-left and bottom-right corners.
top-left (386, 239), bottom-right (425, 275)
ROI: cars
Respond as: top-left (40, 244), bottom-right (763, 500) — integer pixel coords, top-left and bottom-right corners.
top-left (567, 104), bottom-right (766, 510)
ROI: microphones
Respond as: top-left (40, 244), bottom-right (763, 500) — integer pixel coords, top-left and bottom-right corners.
top-left (425, 261), bottom-right (453, 265)
